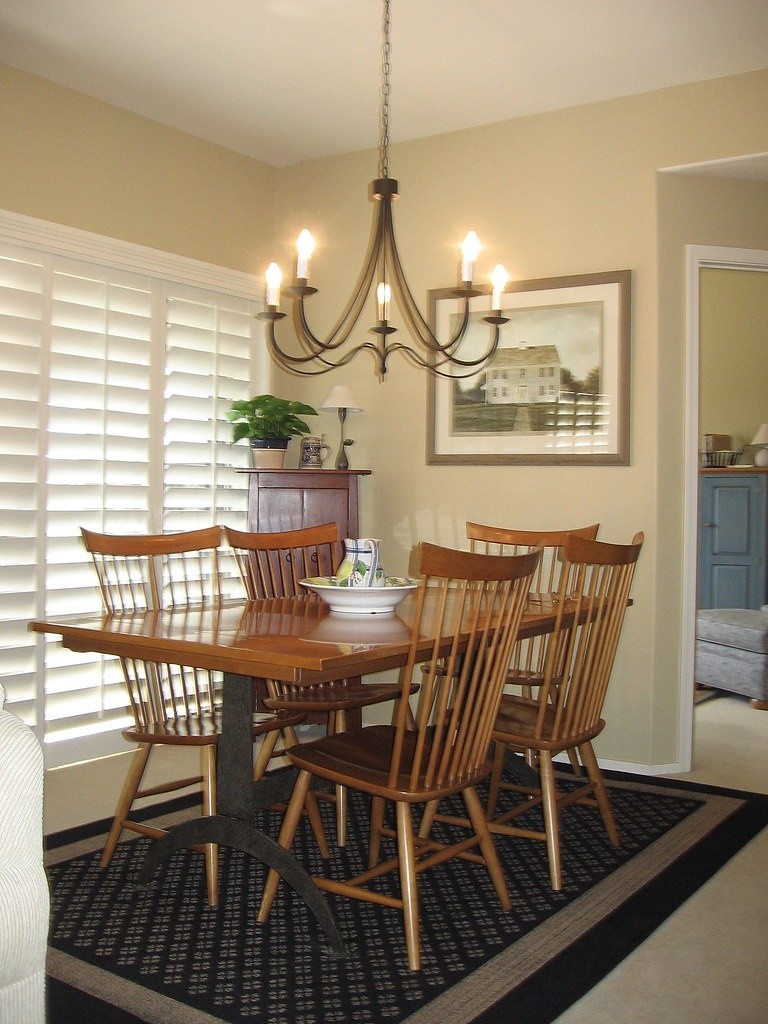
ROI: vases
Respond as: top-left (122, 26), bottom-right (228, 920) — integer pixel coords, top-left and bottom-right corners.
top-left (335, 538), bottom-right (387, 587)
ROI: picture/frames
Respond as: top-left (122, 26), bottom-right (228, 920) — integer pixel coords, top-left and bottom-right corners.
top-left (425, 269), bottom-right (631, 467)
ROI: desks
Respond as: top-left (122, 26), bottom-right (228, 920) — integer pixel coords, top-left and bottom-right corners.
top-left (27, 585), bottom-right (635, 926)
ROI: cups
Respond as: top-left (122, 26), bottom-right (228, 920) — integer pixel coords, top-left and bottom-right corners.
top-left (298, 437), bottom-right (331, 469)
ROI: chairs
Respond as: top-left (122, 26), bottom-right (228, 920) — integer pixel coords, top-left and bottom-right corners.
top-left (223, 525), bottom-right (422, 859)
top-left (256, 541), bottom-right (545, 972)
top-left (79, 525), bottom-right (332, 909)
top-left (420, 520), bottom-right (603, 816)
top-left (418, 531), bottom-right (645, 889)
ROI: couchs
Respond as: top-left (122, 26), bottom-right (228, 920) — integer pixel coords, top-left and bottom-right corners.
top-left (694, 605), bottom-right (768, 710)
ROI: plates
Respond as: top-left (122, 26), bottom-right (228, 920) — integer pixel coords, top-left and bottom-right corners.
top-left (299, 576), bottom-right (420, 614)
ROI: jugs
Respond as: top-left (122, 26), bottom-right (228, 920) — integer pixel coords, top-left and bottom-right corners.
top-left (338, 538), bottom-right (384, 586)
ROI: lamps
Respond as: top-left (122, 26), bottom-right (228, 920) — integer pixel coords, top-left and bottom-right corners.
top-left (254, 0), bottom-right (512, 383)
top-left (319, 385), bottom-right (364, 471)
top-left (745, 423), bottom-right (768, 466)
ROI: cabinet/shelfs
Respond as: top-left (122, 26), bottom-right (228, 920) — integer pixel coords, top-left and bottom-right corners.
top-left (696, 467), bottom-right (768, 610)
top-left (233, 468), bottom-right (372, 725)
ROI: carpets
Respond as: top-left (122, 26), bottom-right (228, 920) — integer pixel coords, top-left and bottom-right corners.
top-left (43, 755), bottom-right (768, 1024)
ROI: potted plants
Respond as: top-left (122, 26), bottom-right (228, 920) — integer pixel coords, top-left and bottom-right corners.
top-left (224, 395), bottom-right (319, 470)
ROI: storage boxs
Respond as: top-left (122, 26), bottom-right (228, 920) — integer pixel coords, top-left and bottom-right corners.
top-left (702, 434), bottom-right (731, 469)
